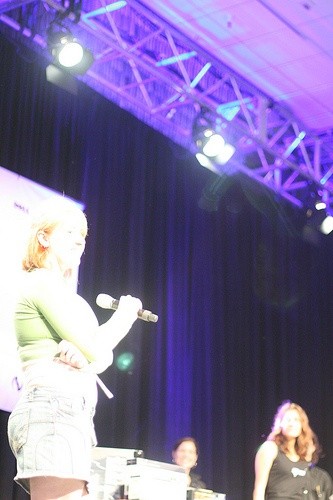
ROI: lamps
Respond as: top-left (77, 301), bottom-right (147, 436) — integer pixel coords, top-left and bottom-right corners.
top-left (194, 127), bottom-right (224, 158)
top-left (45, 22), bottom-right (88, 70)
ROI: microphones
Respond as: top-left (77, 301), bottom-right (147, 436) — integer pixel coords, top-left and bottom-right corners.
top-left (96, 293), bottom-right (159, 323)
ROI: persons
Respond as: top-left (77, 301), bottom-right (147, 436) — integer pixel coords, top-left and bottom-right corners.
top-left (167, 436), bottom-right (208, 500)
top-left (7, 203), bottom-right (142, 500)
top-left (252, 402), bottom-right (321, 500)
top-left (301, 428), bottom-right (332, 500)
